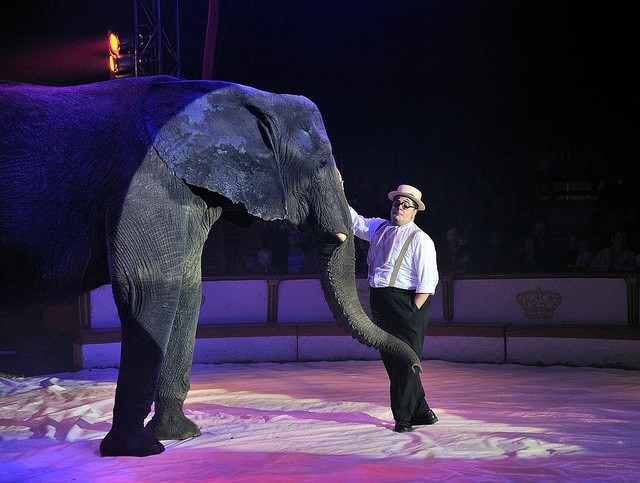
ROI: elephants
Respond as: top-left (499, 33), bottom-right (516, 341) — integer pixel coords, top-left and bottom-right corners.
top-left (0, 74), bottom-right (423, 457)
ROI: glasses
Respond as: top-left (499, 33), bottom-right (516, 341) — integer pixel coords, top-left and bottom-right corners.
top-left (393, 199), bottom-right (417, 210)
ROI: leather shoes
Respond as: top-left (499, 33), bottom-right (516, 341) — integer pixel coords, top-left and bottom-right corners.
top-left (410, 410), bottom-right (438, 424)
top-left (394, 421), bottom-right (412, 433)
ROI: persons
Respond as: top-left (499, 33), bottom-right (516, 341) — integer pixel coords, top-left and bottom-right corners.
top-left (527, 222), bottom-right (552, 256)
top-left (511, 236), bottom-right (545, 273)
top-left (438, 224), bottom-right (460, 272)
top-left (565, 238), bottom-right (596, 268)
top-left (338, 170), bottom-right (440, 432)
top-left (287, 229), bottom-right (304, 274)
top-left (588, 228), bottom-right (639, 272)
top-left (482, 224), bottom-right (511, 275)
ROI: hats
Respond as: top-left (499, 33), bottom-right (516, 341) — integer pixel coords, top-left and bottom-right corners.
top-left (388, 185), bottom-right (425, 211)
